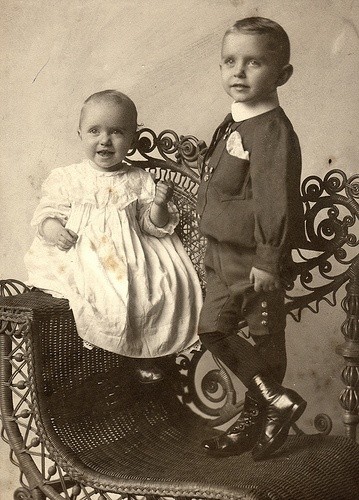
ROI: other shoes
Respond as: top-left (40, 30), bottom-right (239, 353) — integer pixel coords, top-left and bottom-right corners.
top-left (130, 355), bottom-right (171, 383)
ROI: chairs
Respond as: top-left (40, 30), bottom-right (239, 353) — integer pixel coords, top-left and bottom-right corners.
top-left (0, 128), bottom-right (359, 500)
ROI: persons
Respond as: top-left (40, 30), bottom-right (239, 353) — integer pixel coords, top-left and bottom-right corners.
top-left (23, 89), bottom-right (203, 384)
top-left (197, 16), bottom-right (308, 461)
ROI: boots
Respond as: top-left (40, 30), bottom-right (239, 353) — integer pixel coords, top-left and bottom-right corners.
top-left (248, 372), bottom-right (307, 461)
top-left (200, 390), bottom-right (263, 456)
